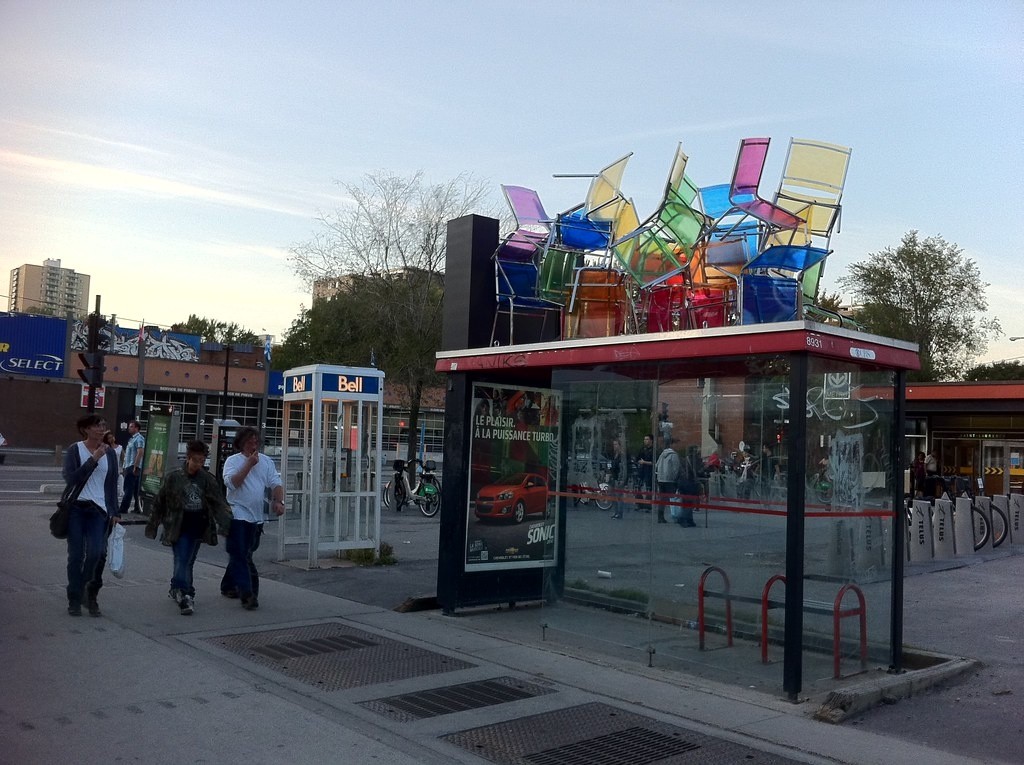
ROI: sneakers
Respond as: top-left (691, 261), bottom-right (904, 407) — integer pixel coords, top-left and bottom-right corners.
top-left (613, 515), bottom-right (623, 520)
top-left (68, 597), bottom-right (82, 616)
top-left (237, 590), bottom-right (258, 610)
top-left (658, 518), bottom-right (668, 524)
top-left (221, 585), bottom-right (239, 598)
top-left (177, 588), bottom-right (194, 615)
top-left (81, 599), bottom-right (101, 617)
top-left (168, 585), bottom-right (195, 600)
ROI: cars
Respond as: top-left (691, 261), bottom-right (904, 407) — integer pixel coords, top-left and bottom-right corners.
top-left (743, 455), bottom-right (788, 484)
top-left (569, 452), bottom-right (611, 473)
top-left (474, 472), bottom-right (552, 524)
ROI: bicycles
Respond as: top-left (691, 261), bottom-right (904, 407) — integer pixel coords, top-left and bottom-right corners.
top-left (382, 457), bottom-right (442, 518)
top-left (814, 468), bottom-right (858, 504)
top-left (570, 476), bottom-right (615, 514)
top-left (902, 474), bottom-right (1009, 552)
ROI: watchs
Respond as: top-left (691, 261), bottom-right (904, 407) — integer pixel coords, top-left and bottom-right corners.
top-left (280, 500), bottom-right (285, 505)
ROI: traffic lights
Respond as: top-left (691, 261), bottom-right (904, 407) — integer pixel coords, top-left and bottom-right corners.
top-left (76, 351), bottom-right (107, 387)
top-left (776, 424), bottom-right (782, 444)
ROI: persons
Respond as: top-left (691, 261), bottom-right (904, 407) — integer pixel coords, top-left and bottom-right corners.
top-left (608, 439), bottom-right (628, 520)
top-left (103, 421), bottom-right (144, 513)
top-left (761, 441), bottom-right (780, 480)
top-left (220, 427), bottom-right (285, 608)
top-left (709, 450), bottom-right (720, 466)
top-left (634, 434), bottom-right (653, 512)
top-left (63, 412), bottom-right (122, 616)
top-left (912, 450), bottom-right (938, 499)
top-left (656, 437), bottom-right (705, 524)
top-left (145, 440), bottom-right (235, 616)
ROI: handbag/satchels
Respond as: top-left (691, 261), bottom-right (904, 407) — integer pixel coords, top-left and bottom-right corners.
top-left (49, 501), bottom-right (71, 539)
top-left (107, 523), bottom-right (126, 579)
top-left (671, 490), bottom-right (684, 519)
